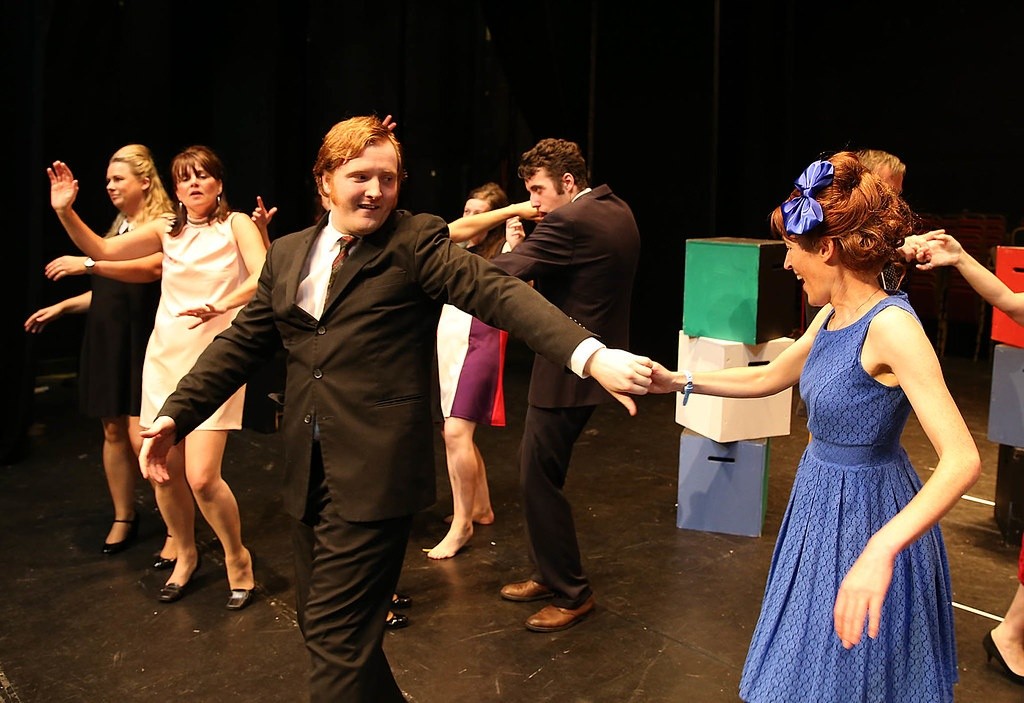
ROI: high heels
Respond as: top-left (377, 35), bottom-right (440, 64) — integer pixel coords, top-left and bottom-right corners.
top-left (982, 630), bottom-right (1024, 685)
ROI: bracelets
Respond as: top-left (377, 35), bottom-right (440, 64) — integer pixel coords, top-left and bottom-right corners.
top-left (681, 371), bottom-right (694, 406)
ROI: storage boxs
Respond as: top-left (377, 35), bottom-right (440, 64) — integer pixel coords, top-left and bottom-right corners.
top-left (986, 245), bottom-right (1024, 547)
top-left (675, 237), bottom-right (797, 537)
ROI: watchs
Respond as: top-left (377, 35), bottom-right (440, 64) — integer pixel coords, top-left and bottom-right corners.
top-left (84, 258), bottom-right (95, 275)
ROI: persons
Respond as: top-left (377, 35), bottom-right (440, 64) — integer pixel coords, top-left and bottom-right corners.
top-left (24, 142), bottom-right (177, 570)
top-left (47, 145), bottom-right (268, 609)
top-left (647, 152), bottom-right (981, 703)
top-left (252, 196), bottom-right (277, 249)
top-left (382, 114), bottom-right (543, 630)
top-left (427, 182), bottom-right (534, 558)
top-left (139, 117), bottom-right (653, 703)
top-left (854, 150), bottom-right (1024, 683)
top-left (490, 137), bottom-right (640, 632)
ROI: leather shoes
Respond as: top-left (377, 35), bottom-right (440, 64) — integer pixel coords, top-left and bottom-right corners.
top-left (500, 578), bottom-right (557, 602)
top-left (153, 535), bottom-right (177, 570)
top-left (524, 594), bottom-right (596, 632)
top-left (385, 608), bottom-right (408, 629)
top-left (101, 510), bottom-right (138, 555)
top-left (389, 591), bottom-right (412, 609)
top-left (157, 545), bottom-right (201, 603)
top-left (224, 545), bottom-right (256, 611)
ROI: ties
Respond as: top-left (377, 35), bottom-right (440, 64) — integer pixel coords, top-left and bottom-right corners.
top-left (323, 235), bottom-right (361, 311)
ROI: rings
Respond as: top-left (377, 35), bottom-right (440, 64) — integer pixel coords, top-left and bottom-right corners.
top-left (926, 263), bottom-right (932, 269)
